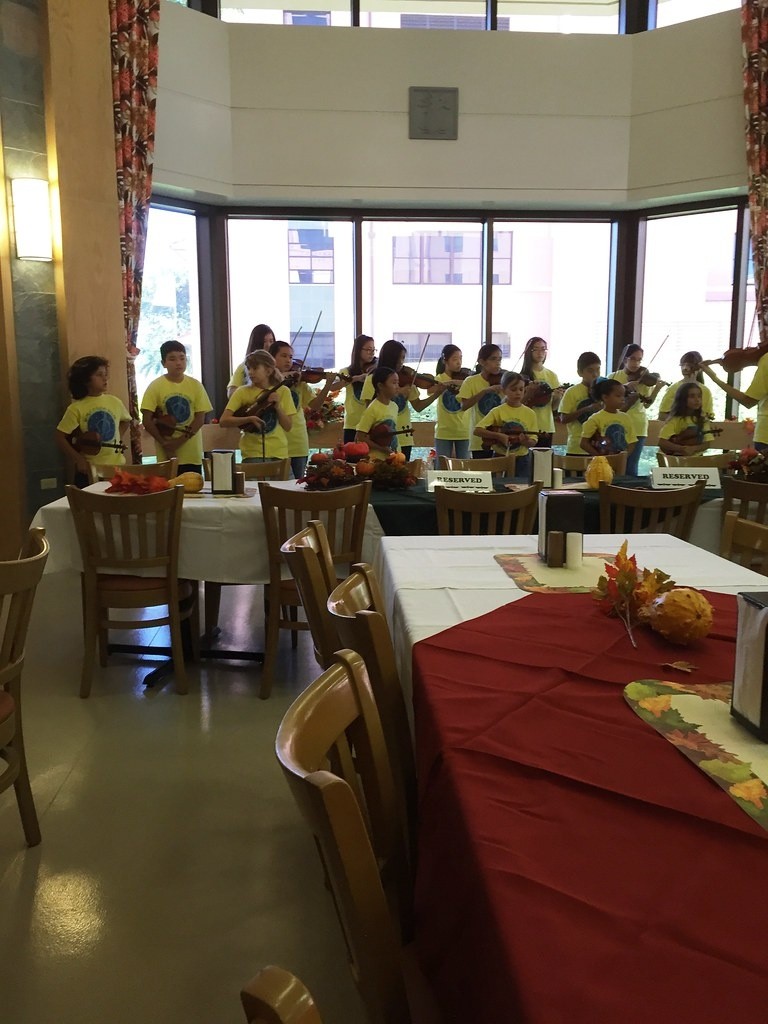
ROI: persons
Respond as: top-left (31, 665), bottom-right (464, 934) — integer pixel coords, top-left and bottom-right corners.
top-left (54, 356), bottom-right (133, 489)
top-left (219, 324), bottom-right (768, 484)
top-left (140, 340), bottom-right (213, 477)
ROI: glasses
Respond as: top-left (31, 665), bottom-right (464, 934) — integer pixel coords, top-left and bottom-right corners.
top-left (361, 348), bottom-right (377, 354)
top-left (530, 349), bottom-right (548, 353)
top-left (625, 356), bottom-right (642, 361)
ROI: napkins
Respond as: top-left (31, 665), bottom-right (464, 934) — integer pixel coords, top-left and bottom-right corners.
top-left (492, 552), bottom-right (644, 594)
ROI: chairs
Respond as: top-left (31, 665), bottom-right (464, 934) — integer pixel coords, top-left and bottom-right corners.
top-left (719, 511), bottom-right (768, 576)
top-left (435, 482), bottom-right (544, 535)
top-left (279, 519), bottom-right (340, 671)
top-left (278, 645), bottom-right (446, 1024)
top-left (328, 562), bottom-right (423, 852)
top-left (64, 482), bottom-right (187, 697)
top-left (93, 420), bottom-right (756, 480)
top-left (257, 480), bottom-right (371, 699)
top-left (721, 476), bottom-right (768, 528)
top-left (599, 480), bottom-right (706, 542)
top-left (0, 525), bottom-right (47, 847)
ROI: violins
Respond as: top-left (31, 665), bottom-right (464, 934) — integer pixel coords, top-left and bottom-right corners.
top-left (284, 357), bottom-right (354, 384)
top-left (659, 411), bottom-right (724, 456)
top-left (575, 366), bottom-right (672, 457)
top-left (446, 367), bottom-right (574, 409)
top-left (362, 356), bottom-right (460, 396)
top-left (64, 428), bottom-right (130, 457)
top-left (480, 424), bottom-right (553, 447)
top-left (690, 338), bottom-right (768, 374)
top-left (151, 406), bottom-right (196, 440)
top-left (353, 422), bottom-right (416, 448)
top-left (231, 376), bottom-right (295, 434)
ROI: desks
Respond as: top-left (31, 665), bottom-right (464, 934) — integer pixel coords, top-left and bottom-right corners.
top-left (375, 529), bottom-right (768, 1024)
top-left (18, 480), bottom-right (768, 683)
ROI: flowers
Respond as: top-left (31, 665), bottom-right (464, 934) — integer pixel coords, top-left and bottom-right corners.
top-left (305, 389), bottom-right (345, 434)
top-left (731, 447), bottom-right (765, 480)
